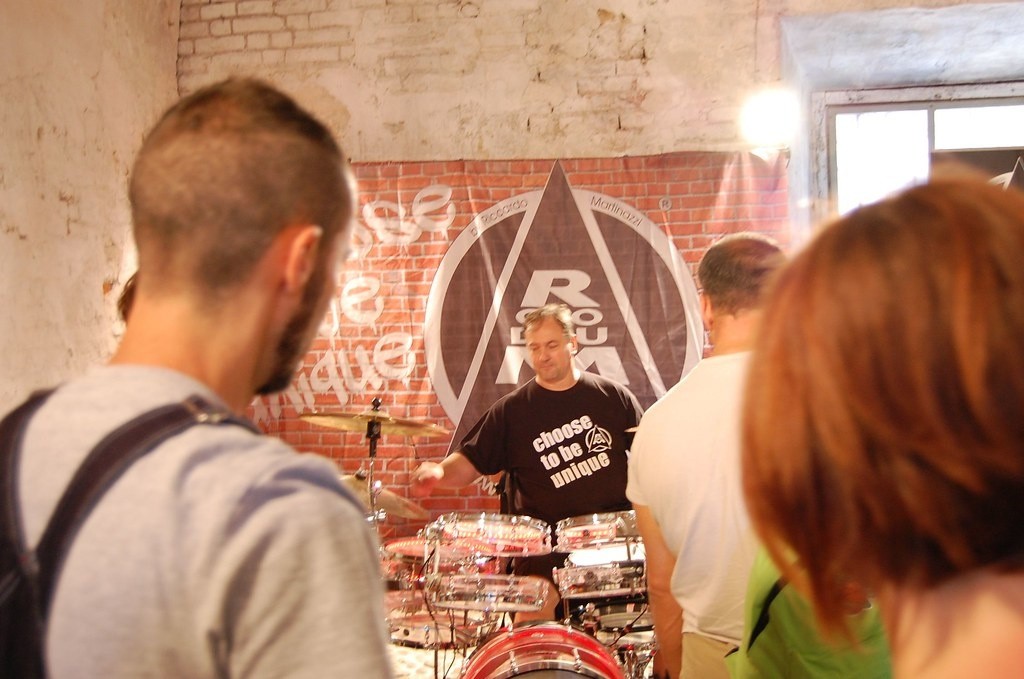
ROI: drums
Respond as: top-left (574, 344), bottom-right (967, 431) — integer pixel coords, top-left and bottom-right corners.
top-left (381, 509), bottom-right (657, 679)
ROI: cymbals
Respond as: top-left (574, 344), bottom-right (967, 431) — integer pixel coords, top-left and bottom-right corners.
top-left (297, 409), bottom-right (449, 522)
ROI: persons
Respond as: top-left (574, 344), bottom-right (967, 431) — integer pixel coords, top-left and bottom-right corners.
top-left (407, 303), bottom-right (644, 622)
top-left (730, 535), bottom-right (892, 678)
top-left (739, 174), bottom-right (1023, 679)
top-left (625, 231), bottom-right (788, 678)
top-left (0, 74), bottom-right (397, 679)
